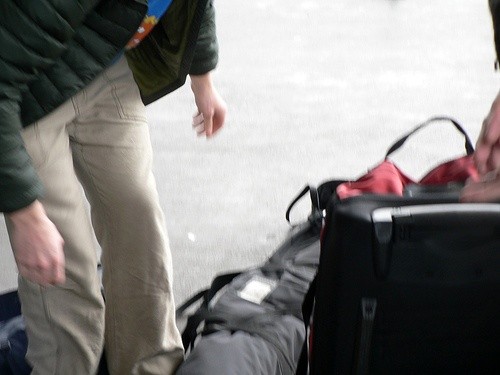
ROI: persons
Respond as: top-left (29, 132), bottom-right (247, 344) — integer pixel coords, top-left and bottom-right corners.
top-left (1, 0), bottom-right (226, 374)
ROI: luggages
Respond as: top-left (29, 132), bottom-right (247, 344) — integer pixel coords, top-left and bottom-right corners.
top-left (294, 193), bottom-right (500, 375)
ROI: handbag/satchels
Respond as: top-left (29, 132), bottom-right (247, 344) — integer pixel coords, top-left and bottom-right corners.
top-left (286, 115), bottom-right (498, 230)
top-left (175, 219), bottom-right (319, 375)
